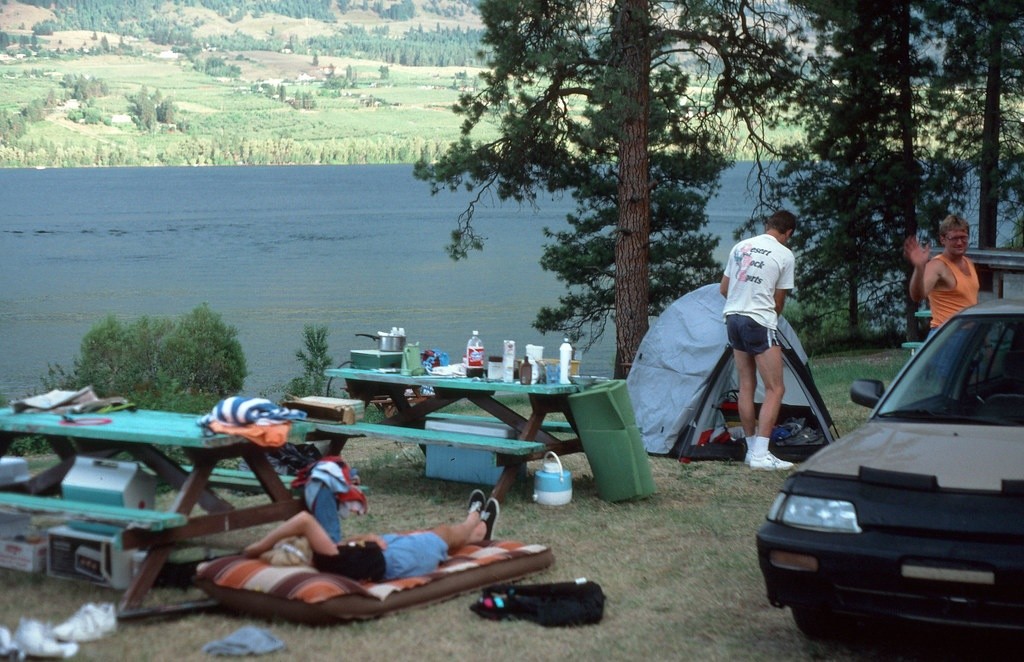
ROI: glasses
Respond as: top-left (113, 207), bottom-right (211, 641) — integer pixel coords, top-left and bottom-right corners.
top-left (943, 236), bottom-right (969, 242)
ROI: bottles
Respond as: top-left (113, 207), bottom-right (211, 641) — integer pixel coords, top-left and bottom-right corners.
top-left (559, 338), bottom-right (572, 384)
top-left (466, 330), bottom-right (484, 378)
top-left (520, 356), bottom-right (531, 385)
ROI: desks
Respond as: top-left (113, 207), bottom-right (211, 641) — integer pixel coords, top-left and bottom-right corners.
top-left (0, 405), bottom-right (341, 625)
top-left (324, 368), bottom-right (610, 507)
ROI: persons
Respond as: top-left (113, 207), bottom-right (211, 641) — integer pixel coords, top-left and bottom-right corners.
top-left (242, 489), bottom-right (500, 582)
top-left (903, 214), bottom-right (988, 395)
top-left (720, 211), bottom-right (797, 472)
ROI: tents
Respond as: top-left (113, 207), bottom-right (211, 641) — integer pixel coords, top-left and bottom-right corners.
top-left (625, 281), bottom-right (841, 461)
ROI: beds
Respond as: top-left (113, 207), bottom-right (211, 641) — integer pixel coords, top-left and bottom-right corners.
top-left (190, 528), bottom-right (555, 624)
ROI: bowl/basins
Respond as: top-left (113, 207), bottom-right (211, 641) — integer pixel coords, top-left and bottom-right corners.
top-left (568, 376), bottom-right (597, 385)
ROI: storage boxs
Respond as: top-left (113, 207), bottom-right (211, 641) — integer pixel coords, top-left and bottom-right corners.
top-left (424, 419), bottom-right (526, 484)
top-left (516, 359), bottom-right (580, 377)
top-left (0, 455), bottom-right (159, 589)
top-left (282, 394), bottom-right (365, 424)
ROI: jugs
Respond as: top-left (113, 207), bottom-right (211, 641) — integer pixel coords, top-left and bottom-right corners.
top-left (400, 345), bottom-right (420, 375)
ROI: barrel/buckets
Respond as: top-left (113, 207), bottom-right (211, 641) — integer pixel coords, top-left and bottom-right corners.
top-left (532, 452), bottom-right (573, 506)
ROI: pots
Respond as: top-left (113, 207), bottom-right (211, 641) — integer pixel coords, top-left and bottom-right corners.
top-left (355, 333), bottom-right (407, 352)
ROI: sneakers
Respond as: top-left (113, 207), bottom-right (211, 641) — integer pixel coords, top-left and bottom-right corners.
top-left (750, 453), bottom-right (794, 471)
top-left (481, 498), bottom-right (500, 546)
top-left (468, 488), bottom-right (486, 517)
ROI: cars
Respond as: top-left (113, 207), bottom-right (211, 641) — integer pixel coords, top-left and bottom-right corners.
top-left (756, 300), bottom-right (1024, 662)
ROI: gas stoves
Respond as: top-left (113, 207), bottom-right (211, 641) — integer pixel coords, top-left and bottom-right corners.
top-left (349, 350), bottom-right (423, 370)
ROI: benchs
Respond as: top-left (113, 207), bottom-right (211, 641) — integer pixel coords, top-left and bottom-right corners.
top-left (319, 422), bottom-right (545, 455)
top-left (140, 463), bottom-right (371, 497)
top-left (0, 491), bottom-right (188, 531)
top-left (425, 412), bottom-right (644, 432)
